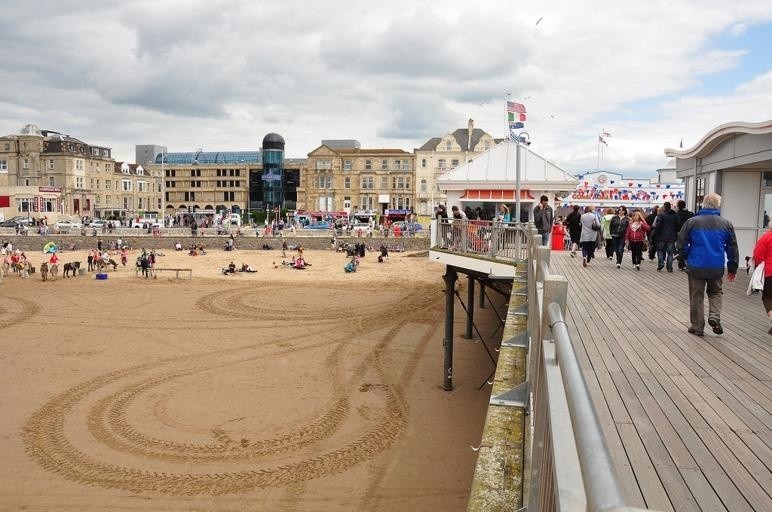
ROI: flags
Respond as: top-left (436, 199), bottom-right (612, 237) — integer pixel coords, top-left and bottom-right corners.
top-left (507, 101), bottom-right (527, 142)
top-left (599, 132), bottom-right (612, 146)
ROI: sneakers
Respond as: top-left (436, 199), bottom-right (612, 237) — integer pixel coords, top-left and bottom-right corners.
top-left (708, 318), bottom-right (723, 334)
top-left (570, 251), bottom-right (576, 258)
top-left (657, 256), bottom-right (687, 272)
top-left (632, 264), bottom-right (640, 270)
top-left (688, 327), bottom-right (703, 336)
top-left (583, 257), bottom-right (587, 267)
top-left (616, 264), bottom-right (620, 269)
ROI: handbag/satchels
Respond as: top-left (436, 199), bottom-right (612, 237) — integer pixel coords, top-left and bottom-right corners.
top-left (642, 241), bottom-right (648, 251)
top-left (592, 223), bottom-right (599, 231)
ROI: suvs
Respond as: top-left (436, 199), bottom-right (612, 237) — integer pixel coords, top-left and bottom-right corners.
top-left (388, 221), bottom-right (422, 234)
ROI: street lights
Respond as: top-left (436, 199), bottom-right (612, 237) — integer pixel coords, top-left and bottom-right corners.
top-left (160, 147), bottom-right (170, 228)
top-left (515, 130), bottom-right (531, 260)
top-left (430, 185), bottom-right (436, 218)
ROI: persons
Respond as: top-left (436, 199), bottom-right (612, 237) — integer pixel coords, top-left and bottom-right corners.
top-left (676, 191), bottom-right (740, 336)
top-left (746, 221), bottom-right (772, 334)
top-left (0, 215), bottom-right (412, 279)
top-left (436, 195), bottom-right (694, 272)
top-left (764, 211), bottom-right (769, 228)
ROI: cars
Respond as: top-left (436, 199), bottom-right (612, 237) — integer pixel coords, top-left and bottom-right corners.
top-left (51, 219), bottom-right (85, 230)
top-left (2, 215), bottom-right (34, 228)
top-left (88, 209), bottom-right (374, 233)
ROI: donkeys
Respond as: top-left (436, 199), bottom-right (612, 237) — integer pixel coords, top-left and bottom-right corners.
top-left (49, 264), bottom-right (60, 281)
top-left (63, 261), bottom-right (82, 278)
top-left (96, 258), bottom-right (119, 272)
top-left (41, 262), bottom-right (48, 282)
